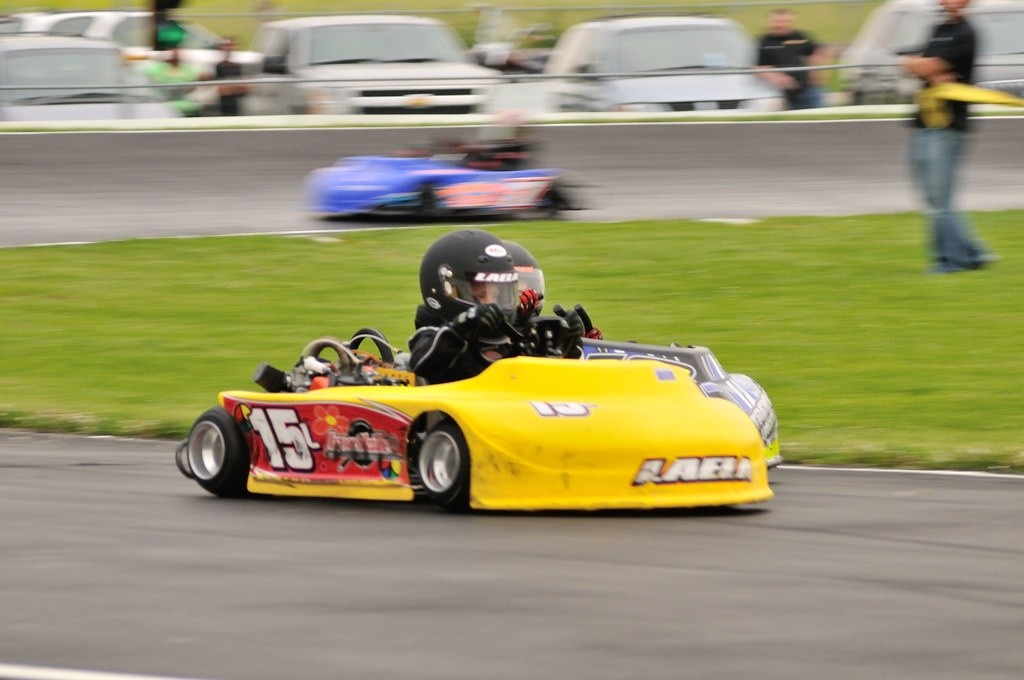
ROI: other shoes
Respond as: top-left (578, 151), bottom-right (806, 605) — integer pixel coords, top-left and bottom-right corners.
top-left (931, 245), bottom-right (988, 273)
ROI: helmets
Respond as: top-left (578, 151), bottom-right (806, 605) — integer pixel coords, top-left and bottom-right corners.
top-left (421, 231), bottom-right (519, 353)
top-left (501, 238), bottom-right (547, 317)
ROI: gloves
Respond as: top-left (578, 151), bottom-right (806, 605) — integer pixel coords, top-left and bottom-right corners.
top-left (516, 288), bottom-right (543, 321)
top-left (565, 311), bottom-right (586, 341)
top-left (584, 328), bottom-right (602, 339)
top-left (452, 301), bottom-right (502, 332)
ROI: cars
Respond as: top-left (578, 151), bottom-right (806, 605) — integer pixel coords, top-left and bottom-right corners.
top-left (0, 35), bottom-right (188, 126)
top-left (21, 11), bottom-right (269, 115)
top-left (546, 17), bottom-right (796, 114)
top-left (837, 1), bottom-right (1024, 109)
top-left (241, 17), bottom-right (523, 113)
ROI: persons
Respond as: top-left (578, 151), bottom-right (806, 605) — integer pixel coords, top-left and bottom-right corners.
top-left (408, 229), bottom-right (603, 386)
top-left (902, 0), bottom-right (994, 275)
top-left (145, 36), bottom-right (254, 116)
top-left (752, 6), bottom-right (837, 111)
